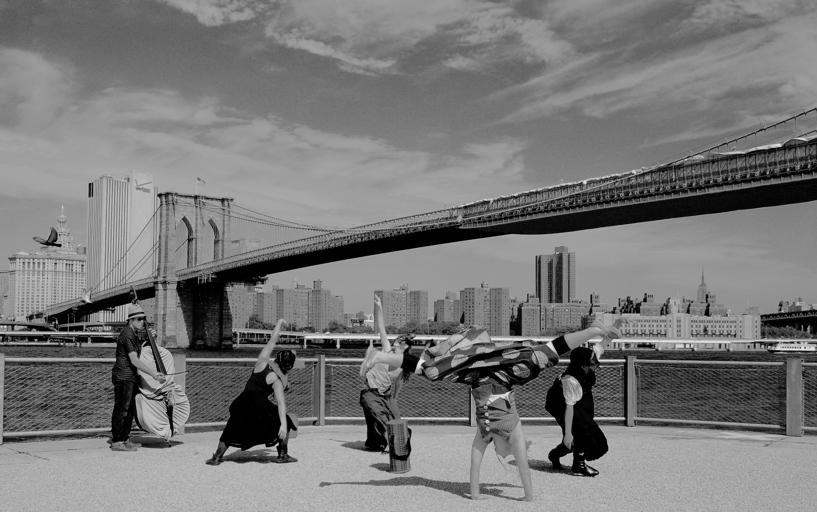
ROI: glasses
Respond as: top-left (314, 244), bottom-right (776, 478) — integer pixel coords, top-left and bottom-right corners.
top-left (138, 317), bottom-right (146, 321)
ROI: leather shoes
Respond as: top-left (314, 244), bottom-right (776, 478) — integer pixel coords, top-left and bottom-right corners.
top-left (113, 438), bottom-right (142, 451)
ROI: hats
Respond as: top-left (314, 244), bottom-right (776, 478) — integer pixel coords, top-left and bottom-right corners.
top-left (125, 307), bottom-right (147, 321)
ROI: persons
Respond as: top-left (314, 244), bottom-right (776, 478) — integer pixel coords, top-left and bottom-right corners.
top-left (355, 317), bottom-right (623, 502)
top-left (107, 304), bottom-right (165, 452)
top-left (357, 293), bottom-right (413, 454)
top-left (205, 318), bottom-right (298, 466)
top-left (542, 318), bottom-right (623, 478)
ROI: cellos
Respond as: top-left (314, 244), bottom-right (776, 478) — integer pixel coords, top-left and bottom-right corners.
top-left (126, 284), bottom-right (191, 435)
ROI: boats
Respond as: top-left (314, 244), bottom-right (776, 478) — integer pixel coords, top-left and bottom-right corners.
top-left (766, 338), bottom-right (817, 353)
top-left (637, 343), bottom-right (654, 348)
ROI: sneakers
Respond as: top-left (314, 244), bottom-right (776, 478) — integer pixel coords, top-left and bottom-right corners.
top-left (359, 345), bottom-right (379, 378)
top-left (591, 318), bottom-right (622, 339)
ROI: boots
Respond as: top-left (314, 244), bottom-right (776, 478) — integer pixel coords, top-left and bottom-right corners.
top-left (549, 444), bottom-right (571, 468)
top-left (276, 445), bottom-right (297, 463)
top-left (572, 452), bottom-right (599, 476)
top-left (207, 448), bottom-right (226, 465)
